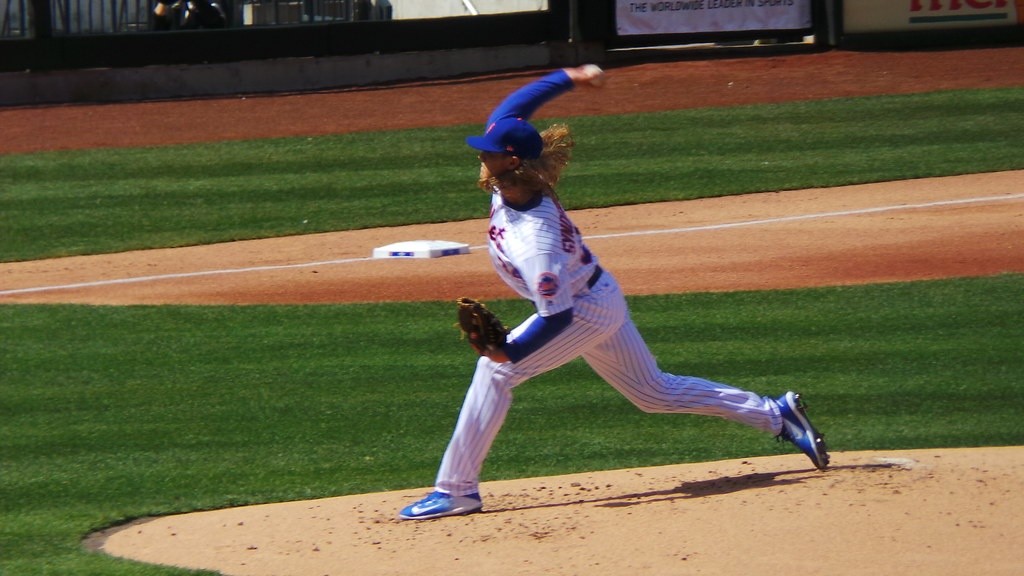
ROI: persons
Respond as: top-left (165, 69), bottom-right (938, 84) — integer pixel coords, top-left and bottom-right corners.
top-left (399, 65), bottom-right (831, 521)
top-left (151, 0), bottom-right (234, 31)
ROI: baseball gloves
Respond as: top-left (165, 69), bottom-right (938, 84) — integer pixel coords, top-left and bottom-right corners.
top-left (458, 296), bottom-right (507, 356)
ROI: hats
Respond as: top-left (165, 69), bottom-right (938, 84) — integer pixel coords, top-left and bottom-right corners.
top-left (466, 116), bottom-right (543, 159)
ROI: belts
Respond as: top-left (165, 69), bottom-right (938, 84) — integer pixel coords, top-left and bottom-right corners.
top-left (587, 265), bottom-right (603, 290)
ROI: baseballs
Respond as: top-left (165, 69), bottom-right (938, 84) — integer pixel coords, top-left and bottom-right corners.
top-left (584, 63), bottom-right (605, 87)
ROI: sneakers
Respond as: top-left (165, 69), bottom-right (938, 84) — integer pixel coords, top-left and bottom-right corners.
top-left (776, 392), bottom-right (830, 469)
top-left (400, 491), bottom-right (482, 519)
